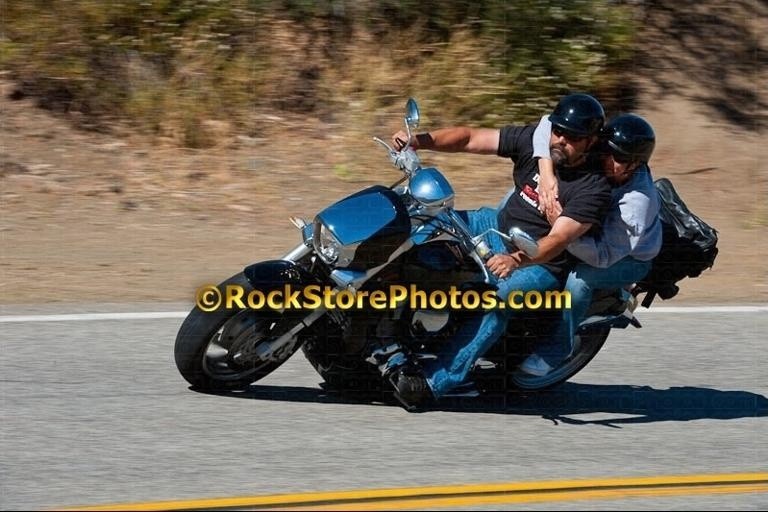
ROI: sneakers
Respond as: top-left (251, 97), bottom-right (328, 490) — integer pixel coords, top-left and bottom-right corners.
top-left (398, 376), bottom-right (436, 403)
top-left (519, 351), bottom-right (555, 377)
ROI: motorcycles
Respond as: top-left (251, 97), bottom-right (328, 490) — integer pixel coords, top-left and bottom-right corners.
top-left (172, 90), bottom-right (641, 395)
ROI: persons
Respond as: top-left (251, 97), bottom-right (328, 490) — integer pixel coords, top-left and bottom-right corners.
top-left (391, 93), bottom-right (662, 410)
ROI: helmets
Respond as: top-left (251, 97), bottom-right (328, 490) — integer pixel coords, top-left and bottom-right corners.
top-left (548, 94), bottom-right (656, 164)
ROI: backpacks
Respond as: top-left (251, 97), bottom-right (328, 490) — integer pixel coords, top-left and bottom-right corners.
top-left (636, 177), bottom-right (719, 300)
